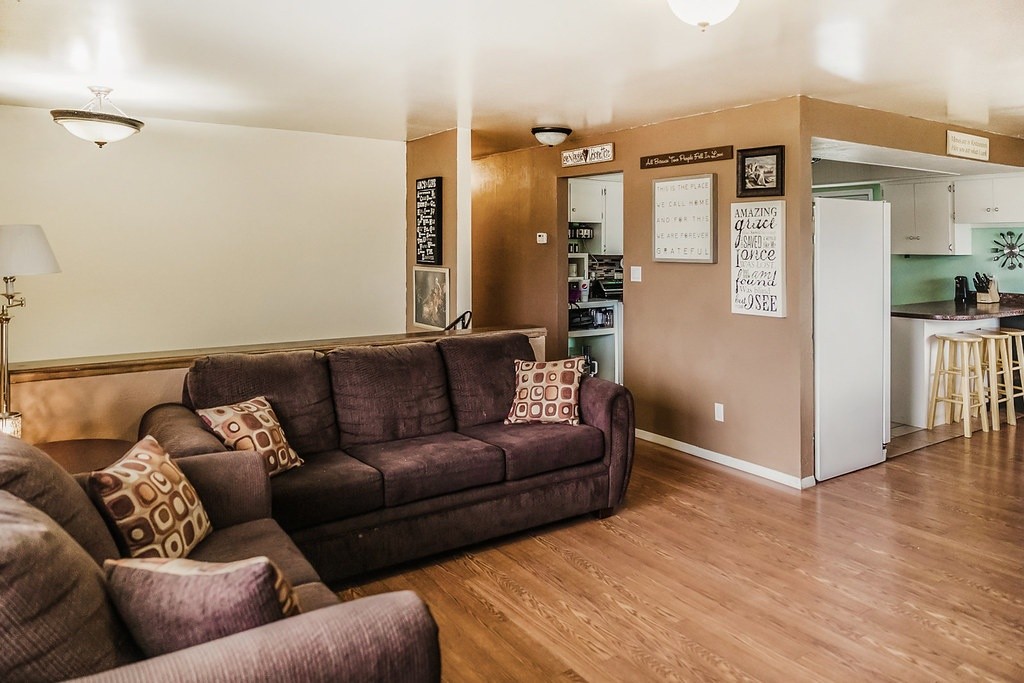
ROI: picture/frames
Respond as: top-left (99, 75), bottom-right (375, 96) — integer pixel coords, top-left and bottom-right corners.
top-left (412, 265), bottom-right (450, 331)
top-left (736, 145), bottom-right (786, 198)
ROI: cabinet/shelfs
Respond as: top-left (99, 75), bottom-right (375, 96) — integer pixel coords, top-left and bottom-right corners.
top-left (880, 171), bottom-right (1024, 256)
top-left (568, 172), bottom-right (623, 255)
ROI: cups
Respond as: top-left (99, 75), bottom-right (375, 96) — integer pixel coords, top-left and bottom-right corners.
top-left (595, 313), bottom-right (603, 324)
top-left (579, 279), bottom-right (589, 301)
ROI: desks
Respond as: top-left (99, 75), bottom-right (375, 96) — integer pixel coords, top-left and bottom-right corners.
top-left (891, 300), bottom-right (1024, 431)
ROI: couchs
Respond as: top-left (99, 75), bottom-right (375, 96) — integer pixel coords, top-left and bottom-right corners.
top-left (0, 333), bottom-right (635, 683)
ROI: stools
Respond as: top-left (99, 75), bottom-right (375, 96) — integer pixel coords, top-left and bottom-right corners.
top-left (927, 326), bottom-right (1024, 438)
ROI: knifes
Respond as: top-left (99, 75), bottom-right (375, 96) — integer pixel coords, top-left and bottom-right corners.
top-left (972, 272), bottom-right (989, 293)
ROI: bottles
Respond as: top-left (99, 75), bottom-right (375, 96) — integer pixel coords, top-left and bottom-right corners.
top-left (568, 259), bottom-right (577, 277)
top-left (568, 243), bottom-right (573, 253)
top-left (574, 242), bottom-right (578, 252)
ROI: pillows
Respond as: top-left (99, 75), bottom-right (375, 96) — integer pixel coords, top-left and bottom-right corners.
top-left (103, 555), bottom-right (301, 659)
top-left (504, 355), bottom-right (588, 427)
top-left (89, 435), bottom-right (213, 559)
top-left (197, 395), bottom-right (306, 474)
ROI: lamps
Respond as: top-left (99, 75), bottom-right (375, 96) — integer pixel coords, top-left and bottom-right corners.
top-left (531, 126), bottom-right (573, 147)
top-left (667, 0), bottom-right (739, 32)
top-left (0, 225), bottom-right (61, 438)
top-left (51, 85), bottom-right (144, 148)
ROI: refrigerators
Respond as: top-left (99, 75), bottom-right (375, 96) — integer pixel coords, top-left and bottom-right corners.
top-left (814, 198), bottom-right (890, 481)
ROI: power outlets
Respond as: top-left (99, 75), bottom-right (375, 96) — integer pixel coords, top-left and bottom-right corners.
top-left (537, 233), bottom-right (547, 243)
top-left (715, 403), bottom-right (724, 422)
